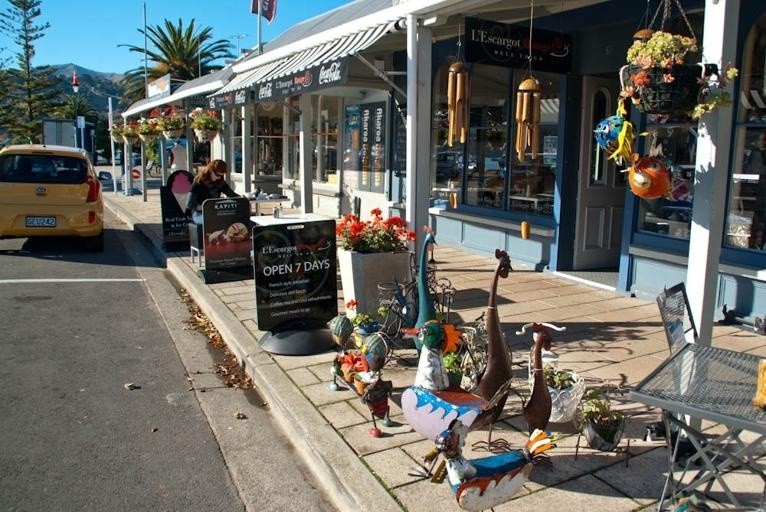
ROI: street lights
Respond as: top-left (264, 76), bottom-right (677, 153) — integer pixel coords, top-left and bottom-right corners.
top-left (230, 32), bottom-right (249, 58)
top-left (71, 69), bottom-right (80, 117)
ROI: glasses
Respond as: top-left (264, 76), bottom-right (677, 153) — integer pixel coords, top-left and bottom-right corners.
top-left (213, 170), bottom-right (222, 177)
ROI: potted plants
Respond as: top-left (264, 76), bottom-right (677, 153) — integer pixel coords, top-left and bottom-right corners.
top-left (531, 367), bottom-right (586, 422)
top-left (578, 389), bottom-right (625, 452)
top-left (443, 353), bottom-right (463, 387)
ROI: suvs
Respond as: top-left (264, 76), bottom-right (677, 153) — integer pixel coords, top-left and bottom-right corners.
top-left (435, 150), bottom-right (554, 215)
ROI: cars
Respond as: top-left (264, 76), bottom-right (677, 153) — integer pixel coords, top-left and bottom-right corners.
top-left (316, 138), bottom-right (397, 176)
top-left (0, 143), bottom-right (105, 251)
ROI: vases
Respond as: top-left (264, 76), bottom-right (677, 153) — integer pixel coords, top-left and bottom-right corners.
top-left (623, 64), bottom-right (716, 114)
top-left (337, 249), bottom-right (410, 336)
top-left (113, 129), bottom-right (216, 145)
top-left (353, 326), bottom-right (377, 347)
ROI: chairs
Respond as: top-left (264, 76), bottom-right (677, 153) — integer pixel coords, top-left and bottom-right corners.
top-left (656, 282), bottom-right (704, 355)
top-left (185, 208), bottom-right (204, 267)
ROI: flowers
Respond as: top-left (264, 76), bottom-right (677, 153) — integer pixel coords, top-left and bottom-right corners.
top-left (108, 108), bottom-right (221, 136)
top-left (346, 301), bottom-right (389, 327)
top-left (616, 32), bottom-right (738, 117)
top-left (335, 208), bottom-right (408, 254)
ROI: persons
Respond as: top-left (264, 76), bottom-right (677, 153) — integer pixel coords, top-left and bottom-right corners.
top-left (183, 160), bottom-right (243, 228)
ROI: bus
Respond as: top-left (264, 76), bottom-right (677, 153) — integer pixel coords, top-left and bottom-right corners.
top-left (90, 148), bottom-right (161, 180)
